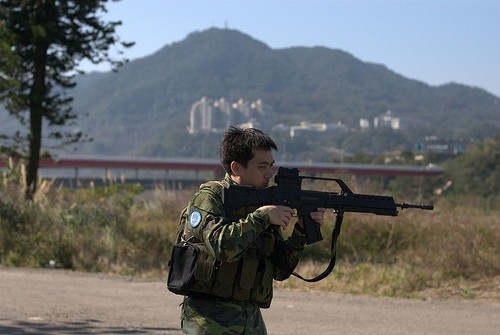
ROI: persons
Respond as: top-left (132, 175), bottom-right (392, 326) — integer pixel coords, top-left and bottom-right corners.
top-left (174, 125), bottom-right (324, 335)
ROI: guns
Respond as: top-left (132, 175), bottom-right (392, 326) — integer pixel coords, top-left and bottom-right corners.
top-left (220, 166), bottom-right (434, 245)
